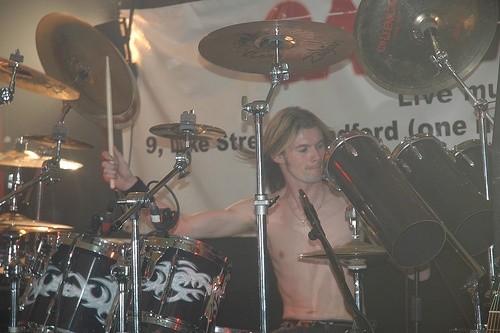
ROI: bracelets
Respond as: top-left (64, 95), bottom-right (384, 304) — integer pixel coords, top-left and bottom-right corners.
top-left (122, 176), bottom-right (150, 198)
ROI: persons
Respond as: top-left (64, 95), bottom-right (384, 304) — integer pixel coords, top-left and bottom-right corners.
top-left (102, 107), bottom-right (433, 333)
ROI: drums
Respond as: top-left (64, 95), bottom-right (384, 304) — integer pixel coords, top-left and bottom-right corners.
top-left (127, 233), bottom-right (231, 333)
top-left (322, 126), bottom-right (500, 267)
top-left (28, 234), bottom-right (124, 333)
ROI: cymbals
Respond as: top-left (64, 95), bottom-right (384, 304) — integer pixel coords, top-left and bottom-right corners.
top-left (24, 135), bottom-right (95, 150)
top-left (149, 123), bottom-right (224, 143)
top-left (0, 150), bottom-right (85, 170)
top-left (0, 213), bottom-right (75, 239)
top-left (0, 58), bottom-right (80, 101)
top-left (297, 236), bottom-right (387, 265)
top-left (35, 13), bottom-right (141, 131)
top-left (197, 21), bottom-right (356, 75)
top-left (354, 0), bottom-right (499, 95)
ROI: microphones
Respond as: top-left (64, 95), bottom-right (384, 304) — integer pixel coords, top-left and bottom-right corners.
top-left (147, 191), bottom-right (169, 236)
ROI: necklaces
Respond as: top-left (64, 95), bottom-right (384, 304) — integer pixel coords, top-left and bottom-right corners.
top-left (284, 179), bottom-right (327, 224)
